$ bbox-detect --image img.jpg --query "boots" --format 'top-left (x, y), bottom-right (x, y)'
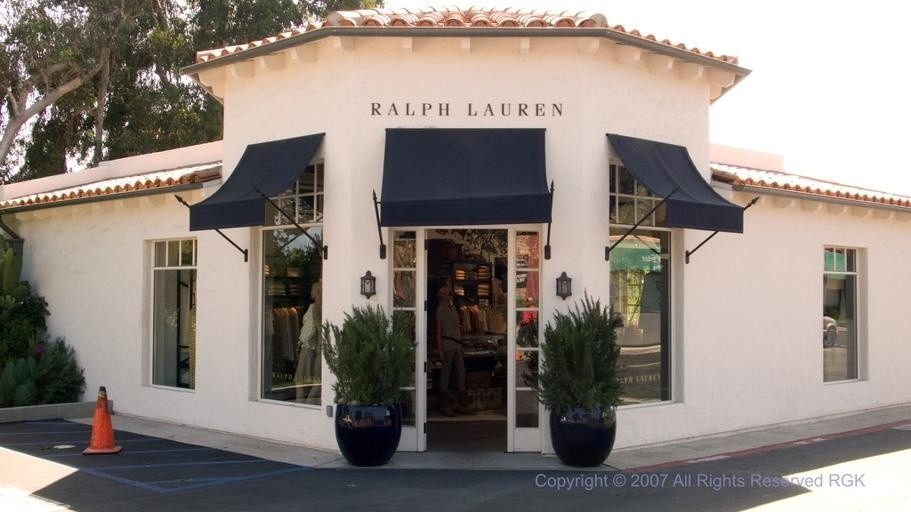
top-left (457, 391), bottom-right (479, 414)
top-left (440, 391), bottom-right (457, 417)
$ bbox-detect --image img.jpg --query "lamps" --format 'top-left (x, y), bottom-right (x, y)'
top-left (556, 271), bottom-right (573, 301)
top-left (359, 270), bottom-right (376, 301)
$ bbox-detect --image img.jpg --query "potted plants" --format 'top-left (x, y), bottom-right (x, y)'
top-left (321, 305), bottom-right (418, 466)
top-left (535, 288), bottom-right (625, 466)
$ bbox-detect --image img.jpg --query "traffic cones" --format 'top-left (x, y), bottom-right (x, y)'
top-left (78, 382), bottom-right (124, 457)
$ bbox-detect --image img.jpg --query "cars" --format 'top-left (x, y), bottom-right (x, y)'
top-left (822, 311), bottom-right (839, 351)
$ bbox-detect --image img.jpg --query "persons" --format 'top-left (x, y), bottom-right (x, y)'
top-left (293, 282), bottom-right (322, 403)
top-left (434, 286), bottom-right (477, 418)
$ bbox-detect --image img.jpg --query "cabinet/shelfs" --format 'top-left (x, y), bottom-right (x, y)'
top-left (262, 253), bottom-right (311, 307)
top-left (453, 258), bottom-right (493, 310)
